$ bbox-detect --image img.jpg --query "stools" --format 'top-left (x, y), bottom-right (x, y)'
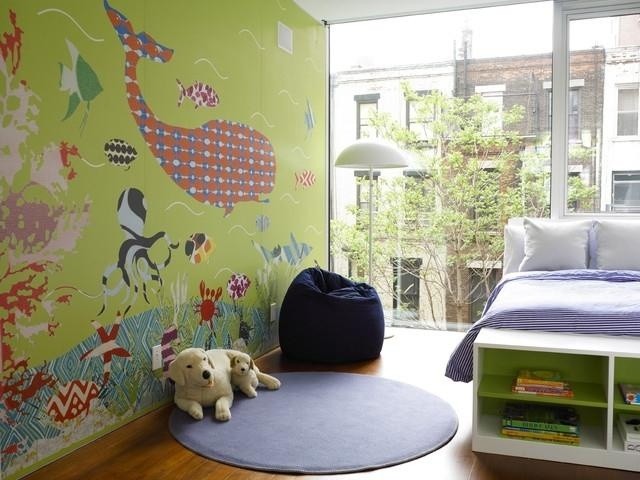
top-left (278, 267), bottom-right (385, 366)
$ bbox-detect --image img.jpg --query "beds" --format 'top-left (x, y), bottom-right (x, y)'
top-left (444, 217), bottom-right (639, 385)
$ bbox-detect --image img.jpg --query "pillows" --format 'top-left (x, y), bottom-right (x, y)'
top-left (518, 217), bottom-right (596, 272)
top-left (589, 218), bottom-right (640, 271)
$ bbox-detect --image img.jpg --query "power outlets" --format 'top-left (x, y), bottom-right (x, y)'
top-left (151, 344), bottom-right (162, 371)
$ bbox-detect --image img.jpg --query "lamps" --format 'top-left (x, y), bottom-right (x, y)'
top-left (333, 142), bottom-right (410, 285)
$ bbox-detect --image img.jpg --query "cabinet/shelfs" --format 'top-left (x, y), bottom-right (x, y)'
top-left (470, 327), bottom-right (495, 468)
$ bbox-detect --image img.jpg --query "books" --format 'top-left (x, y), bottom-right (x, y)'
top-left (615, 383), bottom-right (640, 453)
top-left (499, 367), bottom-right (583, 447)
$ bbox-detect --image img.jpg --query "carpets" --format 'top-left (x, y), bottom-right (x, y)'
top-left (166, 370), bottom-right (459, 475)
